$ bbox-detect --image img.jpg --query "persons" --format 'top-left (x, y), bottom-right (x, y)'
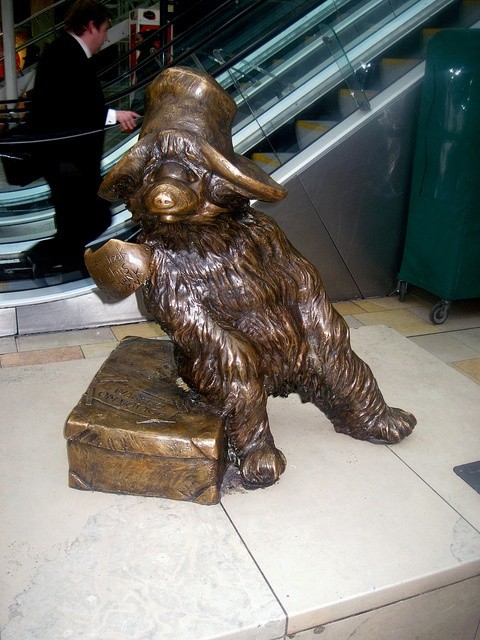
top-left (18, 1), bottom-right (143, 287)
top-left (41, 42), bottom-right (51, 54)
top-left (22, 44), bottom-right (41, 70)
top-left (130, 29), bottom-right (163, 130)
top-left (89, 43), bottom-right (120, 81)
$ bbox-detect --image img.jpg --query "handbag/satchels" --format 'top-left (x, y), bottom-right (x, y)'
top-left (0, 119), bottom-right (44, 186)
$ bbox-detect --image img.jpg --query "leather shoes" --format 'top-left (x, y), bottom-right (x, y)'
top-left (18, 251), bottom-right (49, 289)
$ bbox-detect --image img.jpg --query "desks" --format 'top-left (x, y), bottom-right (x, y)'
top-left (1, 324), bottom-right (479, 640)
